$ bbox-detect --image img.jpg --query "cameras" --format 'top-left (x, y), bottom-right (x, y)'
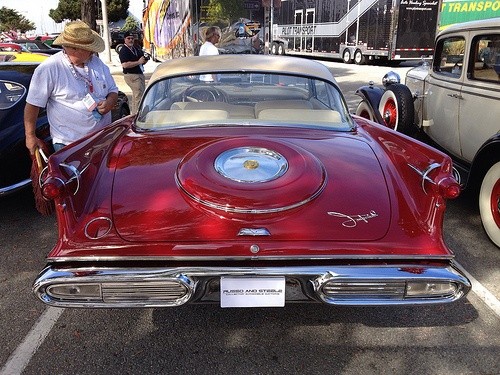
top-left (143, 53), bottom-right (150, 60)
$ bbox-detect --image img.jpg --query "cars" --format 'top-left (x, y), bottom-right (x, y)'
top-left (352, 17), bottom-right (500, 251)
top-left (0, 36), bottom-right (130, 200)
top-left (29, 52), bottom-right (472, 311)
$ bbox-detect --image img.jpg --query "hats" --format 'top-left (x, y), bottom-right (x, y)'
top-left (53, 21), bottom-right (105, 52)
top-left (124, 30), bottom-right (134, 38)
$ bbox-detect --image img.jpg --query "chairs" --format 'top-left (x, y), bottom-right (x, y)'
top-left (474, 68), bottom-right (500, 83)
top-left (257, 108), bottom-right (342, 123)
top-left (145, 110), bottom-right (230, 123)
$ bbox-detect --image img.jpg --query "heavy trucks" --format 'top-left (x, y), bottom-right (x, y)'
top-left (257, 0), bottom-right (443, 65)
top-left (143, 0), bottom-right (264, 63)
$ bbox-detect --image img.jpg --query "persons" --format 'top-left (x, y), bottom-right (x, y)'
top-left (199, 26), bottom-right (221, 84)
top-left (119, 30), bottom-right (150, 116)
top-left (24, 18), bottom-right (119, 178)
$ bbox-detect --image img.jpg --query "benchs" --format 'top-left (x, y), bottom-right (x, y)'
top-left (171, 100), bottom-right (313, 120)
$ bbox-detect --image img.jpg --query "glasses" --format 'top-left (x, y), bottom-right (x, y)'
top-left (127, 38), bottom-right (134, 40)
top-left (215, 34), bottom-right (219, 36)
